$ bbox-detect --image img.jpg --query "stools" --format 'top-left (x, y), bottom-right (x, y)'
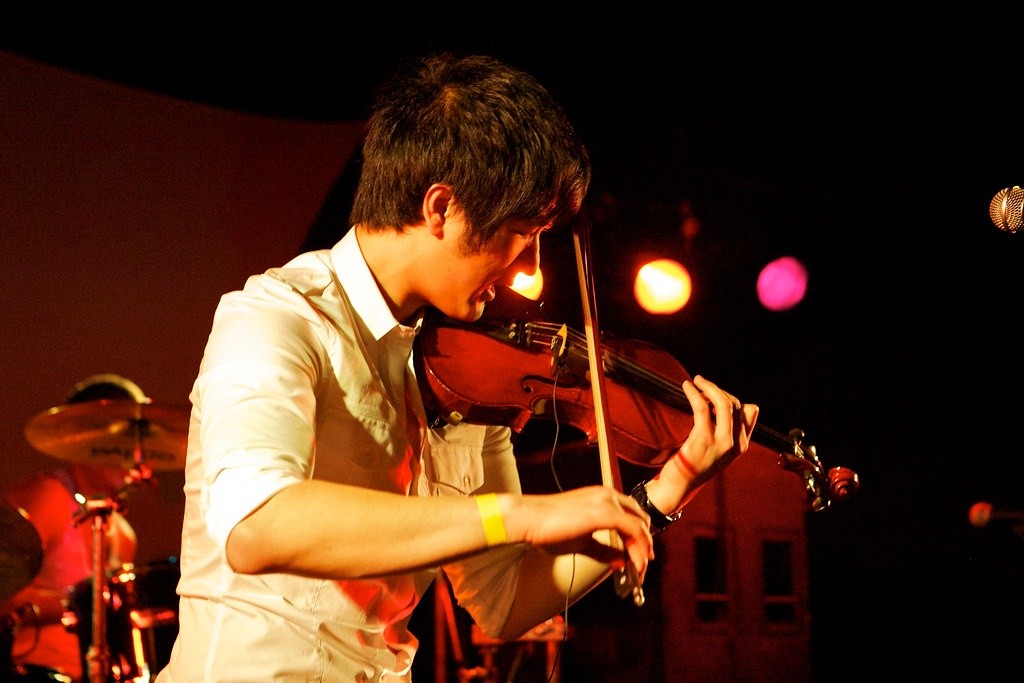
top-left (471, 623), bottom-right (573, 683)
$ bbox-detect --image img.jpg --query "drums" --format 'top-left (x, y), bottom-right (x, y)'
top-left (67, 554), bottom-right (182, 683)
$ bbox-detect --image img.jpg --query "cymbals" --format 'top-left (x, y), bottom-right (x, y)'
top-left (0, 496), bottom-right (44, 606)
top-left (24, 398), bottom-right (197, 472)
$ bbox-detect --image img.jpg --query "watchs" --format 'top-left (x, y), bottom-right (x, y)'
top-left (630, 480), bottom-right (683, 532)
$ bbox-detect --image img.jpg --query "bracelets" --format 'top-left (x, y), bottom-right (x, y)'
top-left (474, 492), bottom-right (506, 549)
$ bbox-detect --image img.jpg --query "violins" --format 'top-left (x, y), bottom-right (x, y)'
top-left (416, 286), bottom-right (865, 514)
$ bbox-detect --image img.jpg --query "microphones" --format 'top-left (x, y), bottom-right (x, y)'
top-left (990, 186), bottom-right (1024, 233)
top-left (968, 503), bottom-right (1024, 526)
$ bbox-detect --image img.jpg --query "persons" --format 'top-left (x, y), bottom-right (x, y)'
top-left (152, 52), bottom-right (759, 683)
top-left (0, 374), bottom-right (146, 683)
